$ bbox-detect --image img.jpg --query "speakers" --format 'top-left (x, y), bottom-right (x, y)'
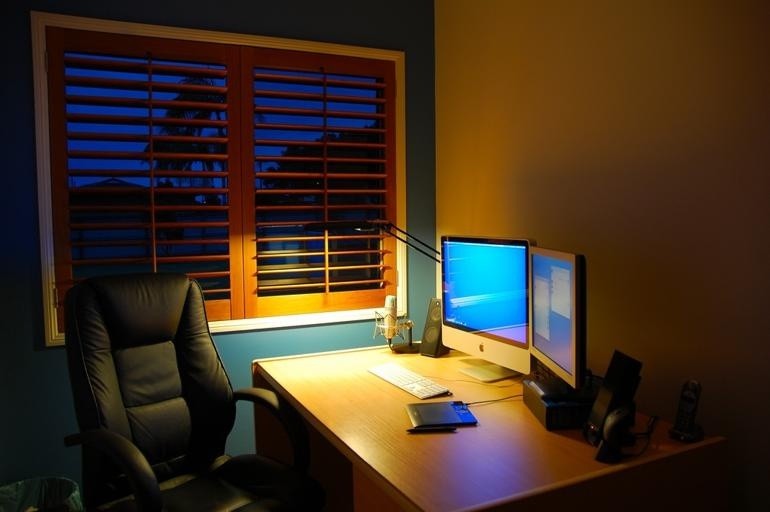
top-left (586, 350), bottom-right (643, 444)
top-left (420, 298), bottom-right (449, 357)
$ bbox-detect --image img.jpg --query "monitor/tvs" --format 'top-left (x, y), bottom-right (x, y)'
top-left (529, 247), bottom-right (589, 399)
top-left (441, 236), bottom-right (536, 382)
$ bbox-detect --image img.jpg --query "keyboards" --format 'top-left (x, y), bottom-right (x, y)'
top-left (369, 363), bottom-right (449, 399)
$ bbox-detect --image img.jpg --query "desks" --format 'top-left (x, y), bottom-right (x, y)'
top-left (251, 343), bottom-right (726, 511)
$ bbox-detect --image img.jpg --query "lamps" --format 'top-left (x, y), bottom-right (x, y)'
top-left (307, 223), bottom-right (440, 264)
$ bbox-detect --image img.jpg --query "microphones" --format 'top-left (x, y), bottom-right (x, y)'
top-left (385, 295), bottom-right (397, 339)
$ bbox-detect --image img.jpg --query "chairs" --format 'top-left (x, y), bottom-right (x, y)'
top-left (64, 276), bottom-right (325, 512)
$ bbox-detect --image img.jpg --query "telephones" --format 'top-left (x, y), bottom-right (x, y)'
top-left (668, 379), bottom-right (706, 442)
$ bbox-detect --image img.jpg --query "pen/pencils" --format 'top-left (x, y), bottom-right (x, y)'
top-left (406, 427), bottom-right (457, 433)
